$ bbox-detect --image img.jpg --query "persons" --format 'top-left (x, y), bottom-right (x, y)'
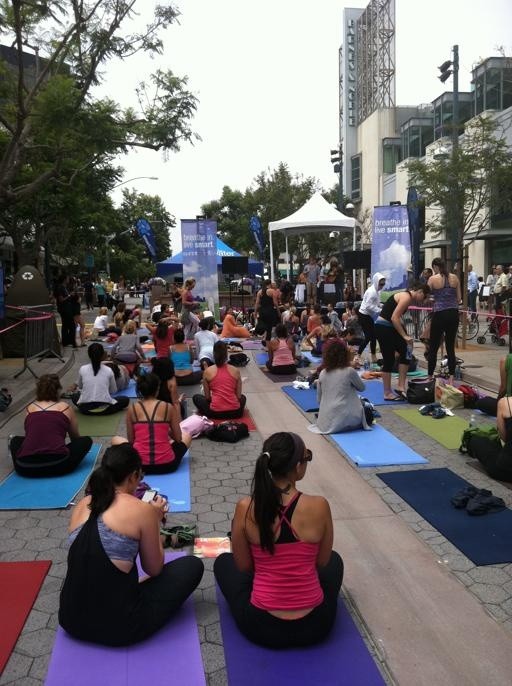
top-left (213, 431), bottom-right (344, 649)
top-left (52, 256), bottom-right (512, 483)
top-left (10, 374), bottom-right (92, 478)
top-left (58, 442), bottom-right (204, 647)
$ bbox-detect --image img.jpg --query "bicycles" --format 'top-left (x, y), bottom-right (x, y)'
top-left (420, 305), bottom-right (479, 341)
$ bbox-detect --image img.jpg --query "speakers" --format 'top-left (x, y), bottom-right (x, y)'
top-left (222, 256), bottom-right (249, 274)
top-left (338, 251), bottom-right (371, 268)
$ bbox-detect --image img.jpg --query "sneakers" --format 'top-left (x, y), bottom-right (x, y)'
top-left (160, 535), bottom-right (170, 549)
top-left (449, 485), bottom-right (478, 510)
top-left (466, 489), bottom-right (505, 515)
top-left (171, 536), bottom-right (184, 548)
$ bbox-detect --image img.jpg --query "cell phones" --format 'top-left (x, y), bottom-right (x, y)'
top-left (141, 490), bottom-right (157, 503)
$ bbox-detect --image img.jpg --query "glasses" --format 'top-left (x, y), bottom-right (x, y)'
top-left (380, 283), bottom-right (385, 286)
top-left (138, 472), bottom-right (145, 480)
top-left (302, 448), bottom-right (312, 462)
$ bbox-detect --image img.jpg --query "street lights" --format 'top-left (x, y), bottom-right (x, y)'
top-left (438, 46), bottom-right (461, 260)
top-left (106, 219), bottom-right (166, 280)
top-left (328, 201), bottom-right (356, 268)
top-left (102, 175), bottom-right (158, 284)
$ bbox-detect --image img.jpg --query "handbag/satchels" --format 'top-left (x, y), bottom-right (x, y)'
top-left (179, 414), bottom-right (214, 439)
top-left (435, 382), bottom-right (464, 409)
top-left (228, 353), bottom-right (250, 367)
top-left (180, 311), bottom-right (190, 325)
top-left (207, 421), bottom-right (249, 443)
top-left (361, 397), bottom-right (376, 426)
top-left (324, 283), bottom-right (336, 293)
top-left (459, 423), bottom-right (501, 453)
top-left (407, 378), bottom-right (436, 404)
top-left (457, 384), bottom-right (479, 409)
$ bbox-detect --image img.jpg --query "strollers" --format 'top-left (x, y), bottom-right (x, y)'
top-left (477, 305), bottom-right (510, 346)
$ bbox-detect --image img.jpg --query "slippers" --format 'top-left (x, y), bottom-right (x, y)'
top-left (384, 397), bottom-right (402, 401)
top-left (394, 390), bottom-right (408, 400)
